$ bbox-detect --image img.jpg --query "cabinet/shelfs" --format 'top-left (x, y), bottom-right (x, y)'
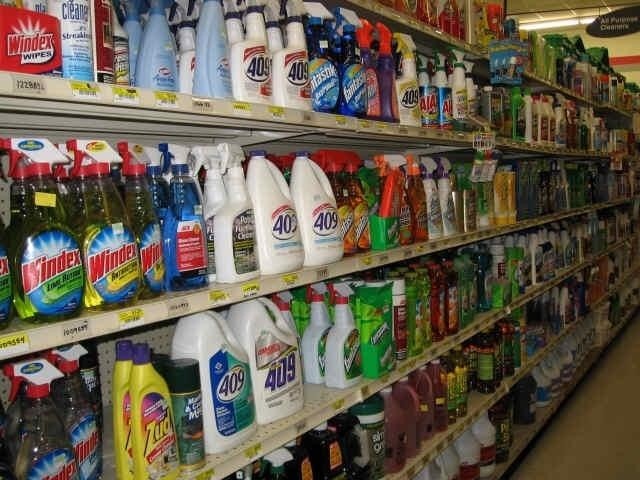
top-left (1, 1), bottom-right (639, 479)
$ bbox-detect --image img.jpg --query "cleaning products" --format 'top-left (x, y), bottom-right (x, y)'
top-left (0, 0), bottom-right (640, 480)
